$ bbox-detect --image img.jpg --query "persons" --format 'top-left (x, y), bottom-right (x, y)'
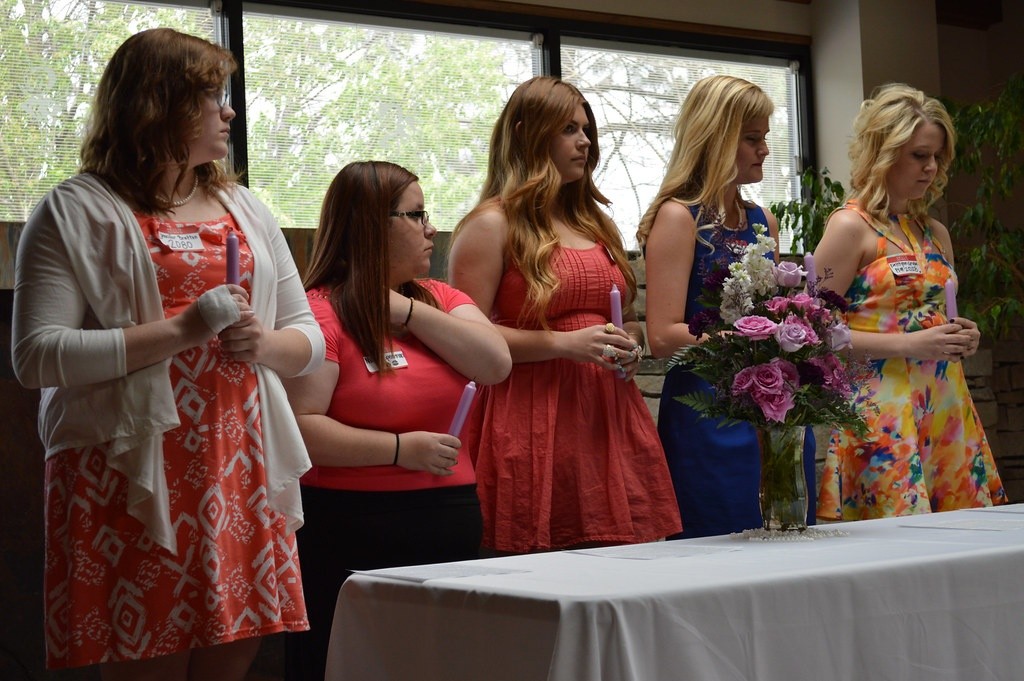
top-left (636, 76), bottom-right (816, 541)
top-left (813, 83), bottom-right (1009, 520)
top-left (448, 77), bottom-right (683, 554)
top-left (284, 161), bottom-right (512, 681)
top-left (11, 30), bottom-right (327, 681)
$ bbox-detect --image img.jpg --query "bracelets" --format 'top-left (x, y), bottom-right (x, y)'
top-left (393, 434), bottom-right (400, 465)
top-left (402, 297), bottom-right (415, 327)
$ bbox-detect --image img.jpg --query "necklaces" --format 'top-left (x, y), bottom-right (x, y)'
top-left (717, 196), bottom-right (743, 232)
top-left (154, 170), bottom-right (199, 206)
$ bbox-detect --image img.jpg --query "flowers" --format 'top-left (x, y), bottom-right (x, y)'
top-left (663, 221), bottom-right (875, 435)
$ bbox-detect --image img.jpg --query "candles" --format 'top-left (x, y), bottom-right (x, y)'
top-left (225, 225), bottom-right (240, 288)
top-left (944, 274), bottom-right (958, 320)
top-left (802, 246), bottom-right (817, 288)
top-left (325, 503), bottom-right (1023, 681)
top-left (608, 278), bottom-right (624, 331)
top-left (447, 375), bottom-right (477, 438)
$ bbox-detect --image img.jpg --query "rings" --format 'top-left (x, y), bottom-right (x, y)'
top-left (602, 345), bottom-right (615, 358)
top-left (634, 346), bottom-right (644, 363)
top-left (605, 323), bottom-right (615, 333)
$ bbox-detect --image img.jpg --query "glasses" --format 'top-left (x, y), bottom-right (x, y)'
top-left (214, 85), bottom-right (233, 108)
top-left (389, 210), bottom-right (430, 226)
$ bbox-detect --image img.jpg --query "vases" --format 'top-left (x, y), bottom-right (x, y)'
top-left (753, 423), bottom-right (811, 532)
